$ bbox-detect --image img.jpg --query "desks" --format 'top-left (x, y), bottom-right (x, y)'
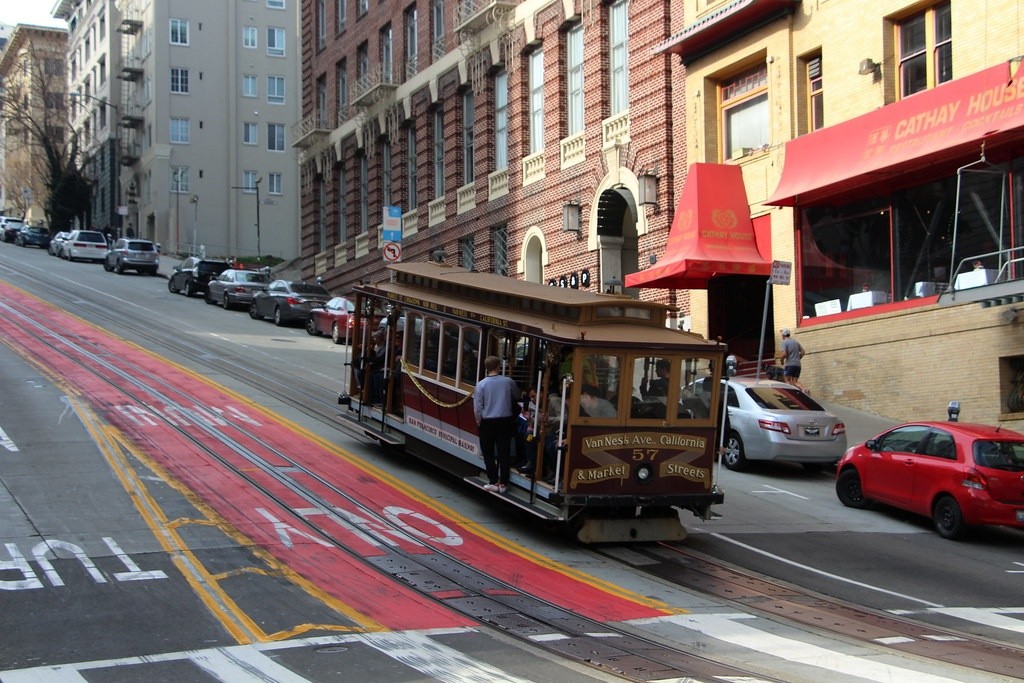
top-left (909, 281), bottom-right (948, 299)
top-left (954, 269), bottom-right (1006, 290)
top-left (847, 291), bottom-right (888, 311)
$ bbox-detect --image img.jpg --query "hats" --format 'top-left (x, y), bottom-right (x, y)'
top-left (782, 329), bottom-right (790, 336)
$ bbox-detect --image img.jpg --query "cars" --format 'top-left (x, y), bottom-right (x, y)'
top-left (834, 420), bottom-right (1024, 538)
top-left (47, 229), bottom-right (108, 262)
top-left (676, 375), bottom-right (849, 471)
top-left (168, 256), bottom-right (423, 345)
top-left (104, 238), bottom-right (160, 274)
top-left (15, 225), bottom-right (51, 248)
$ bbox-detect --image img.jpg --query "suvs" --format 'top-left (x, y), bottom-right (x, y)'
top-left (0, 215), bottom-right (25, 242)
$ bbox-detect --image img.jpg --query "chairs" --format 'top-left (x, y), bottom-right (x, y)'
top-left (933, 439), bottom-right (955, 463)
top-left (934, 281), bottom-right (949, 294)
top-left (886, 289), bottom-right (896, 303)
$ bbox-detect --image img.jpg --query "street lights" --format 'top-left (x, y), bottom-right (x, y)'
top-left (69, 91), bottom-right (120, 228)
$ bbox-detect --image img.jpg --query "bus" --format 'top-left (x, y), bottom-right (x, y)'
top-left (338, 249), bottom-right (738, 545)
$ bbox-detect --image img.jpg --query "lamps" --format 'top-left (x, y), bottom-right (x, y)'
top-left (637, 169), bottom-right (658, 207)
top-left (857, 58), bottom-right (880, 75)
top-left (563, 199), bottom-right (580, 232)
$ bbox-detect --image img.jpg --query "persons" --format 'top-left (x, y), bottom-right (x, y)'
top-left (127, 223), bottom-right (135, 238)
top-left (640, 359), bottom-right (670, 402)
top-left (473, 356), bottom-right (526, 494)
top-left (90, 223), bottom-right (118, 245)
top-left (354, 328), bottom-right (405, 412)
top-left (518, 385), bottom-right (568, 478)
top-left (779, 328), bottom-right (812, 397)
top-left (37, 219), bottom-right (43, 226)
top-left (555, 386), bottom-right (571, 490)
top-left (430, 329), bottom-right (477, 381)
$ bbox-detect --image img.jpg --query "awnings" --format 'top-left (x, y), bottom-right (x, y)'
top-left (625, 162), bottom-right (775, 291)
top-left (648, 0), bottom-right (804, 70)
top-left (765, 55), bottom-right (1024, 209)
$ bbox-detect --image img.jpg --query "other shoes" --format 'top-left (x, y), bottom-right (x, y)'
top-left (483, 483), bottom-right (499, 491)
top-left (805, 389), bottom-right (810, 395)
top-left (521, 460), bottom-right (563, 489)
top-left (499, 485), bottom-right (507, 494)
